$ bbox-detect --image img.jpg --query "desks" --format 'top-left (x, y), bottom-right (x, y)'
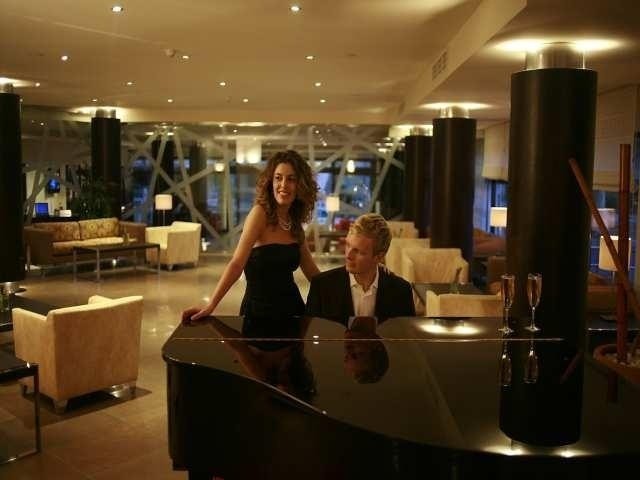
top-left (0, 350), bottom-right (42, 467)
top-left (318, 230), bottom-right (348, 261)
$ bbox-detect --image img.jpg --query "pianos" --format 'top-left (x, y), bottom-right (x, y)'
top-left (161, 316), bottom-right (640, 476)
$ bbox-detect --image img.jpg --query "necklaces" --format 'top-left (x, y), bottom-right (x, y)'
top-left (275, 209), bottom-right (293, 233)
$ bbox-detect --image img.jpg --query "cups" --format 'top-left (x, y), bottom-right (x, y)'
top-left (498, 275), bottom-right (515, 335)
top-left (524, 338), bottom-right (539, 385)
top-left (498, 339), bottom-right (513, 388)
top-left (525, 274), bottom-right (543, 332)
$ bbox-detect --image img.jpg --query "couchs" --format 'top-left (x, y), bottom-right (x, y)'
top-left (386, 221), bottom-right (418, 238)
top-left (385, 238), bottom-right (430, 276)
top-left (424, 289), bottom-right (505, 318)
top-left (401, 247), bottom-right (468, 285)
top-left (11, 293), bottom-right (143, 418)
top-left (144, 220), bottom-right (202, 272)
top-left (24, 216), bottom-right (148, 278)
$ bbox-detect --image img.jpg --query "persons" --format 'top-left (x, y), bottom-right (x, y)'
top-left (179, 317), bottom-right (319, 408)
top-left (181, 149), bottom-right (320, 316)
top-left (305, 213), bottom-right (416, 317)
top-left (317, 317), bottom-right (399, 385)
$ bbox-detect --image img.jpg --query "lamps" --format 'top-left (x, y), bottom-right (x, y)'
top-left (325, 192), bottom-right (340, 212)
top-left (489, 207), bottom-right (507, 231)
top-left (154, 193), bottom-right (173, 226)
top-left (598, 236), bottom-right (631, 285)
